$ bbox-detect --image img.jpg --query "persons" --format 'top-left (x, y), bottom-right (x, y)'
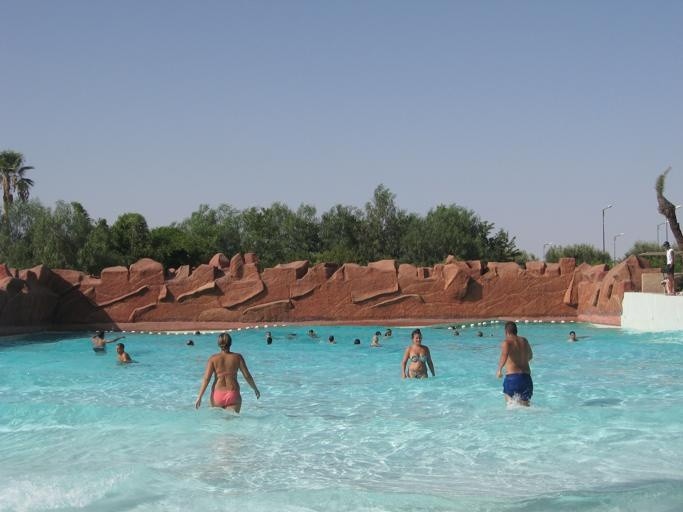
top-left (663, 240), bottom-right (676, 295)
top-left (400, 329), bottom-right (435, 378)
top-left (193, 333), bottom-right (260, 413)
top-left (375, 331), bottom-right (381, 336)
top-left (369, 334), bottom-right (382, 347)
top-left (353, 339), bottom-right (359, 344)
top-left (115, 343), bottom-right (132, 362)
top-left (328, 335), bottom-right (337, 344)
top-left (497, 320), bottom-right (534, 407)
top-left (384, 328), bottom-right (391, 336)
top-left (265, 330), bottom-right (271, 337)
top-left (266, 337), bottom-right (273, 346)
top-left (90, 329), bottom-right (125, 348)
top-left (308, 329), bottom-right (317, 337)
top-left (569, 330), bottom-right (576, 342)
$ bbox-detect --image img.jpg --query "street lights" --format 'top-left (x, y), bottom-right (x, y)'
top-left (542, 242), bottom-right (553, 261)
top-left (656, 221), bottom-right (669, 243)
top-left (666, 205), bottom-right (682, 243)
top-left (613, 232), bottom-right (624, 267)
top-left (602, 204), bottom-right (613, 252)
